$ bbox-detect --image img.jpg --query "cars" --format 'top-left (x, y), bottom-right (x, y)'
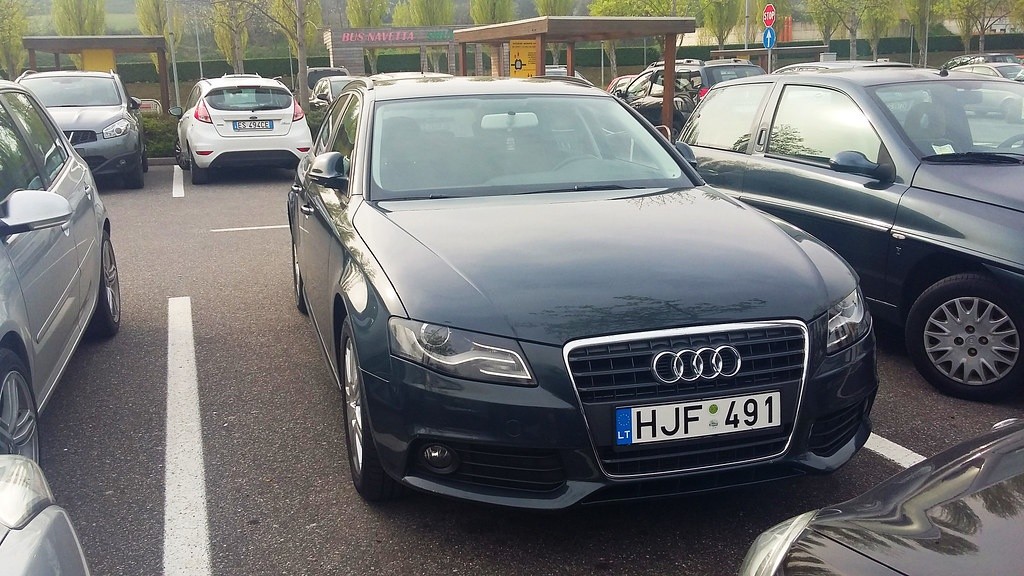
top-left (166, 74), bottom-right (314, 185)
top-left (545, 48), bottom-right (1024, 134)
top-left (308, 75), bottom-right (368, 122)
top-left (295, 76), bottom-right (880, 518)
top-left (1, 79), bottom-right (121, 469)
top-left (675, 63), bottom-right (1023, 402)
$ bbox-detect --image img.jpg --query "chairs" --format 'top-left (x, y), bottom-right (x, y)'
top-left (206, 90), bottom-right (229, 110)
top-left (905, 102), bottom-right (958, 156)
top-left (380, 117), bottom-right (439, 189)
top-left (256, 89), bottom-right (273, 107)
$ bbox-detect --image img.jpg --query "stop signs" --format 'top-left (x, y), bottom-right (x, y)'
top-left (762, 3), bottom-right (776, 28)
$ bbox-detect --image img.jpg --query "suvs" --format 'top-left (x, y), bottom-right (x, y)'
top-left (10, 70), bottom-right (148, 190)
top-left (294, 65), bottom-right (351, 105)
